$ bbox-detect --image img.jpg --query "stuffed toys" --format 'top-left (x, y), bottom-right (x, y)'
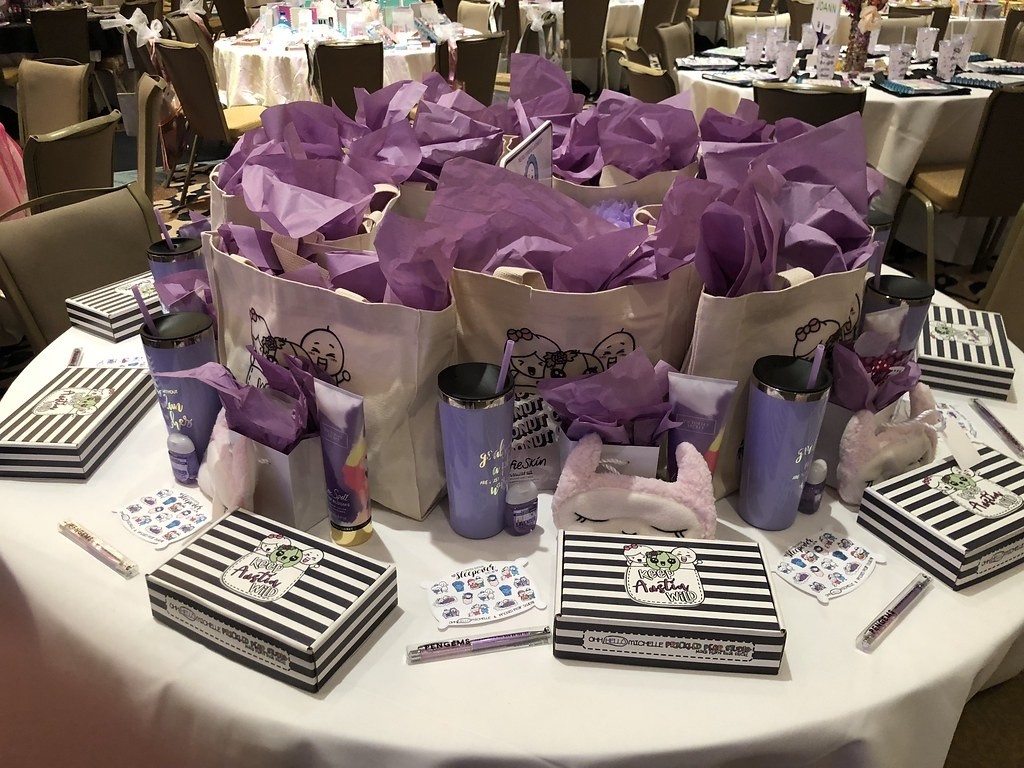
top-left (551, 434), bottom-right (718, 541)
top-left (836, 382), bottom-right (939, 505)
top-left (198, 407), bottom-right (256, 512)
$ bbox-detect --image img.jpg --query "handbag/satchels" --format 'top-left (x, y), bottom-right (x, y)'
top-left (201, 141), bottom-right (866, 533)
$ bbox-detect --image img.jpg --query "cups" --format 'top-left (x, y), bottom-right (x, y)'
top-left (138, 310), bottom-right (223, 465)
top-left (867, 209), bottom-right (893, 274)
top-left (738, 354), bottom-right (832, 531)
top-left (859, 276), bottom-right (936, 356)
top-left (438, 362), bottom-right (515, 539)
top-left (867, 27), bottom-right (974, 81)
top-left (744, 22), bottom-right (839, 79)
top-left (144, 237), bottom-right (205, 281)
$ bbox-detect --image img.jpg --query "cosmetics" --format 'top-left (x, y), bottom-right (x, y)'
top-left (69, 348), bottom-right (83, 366)
top-left (799, 460), bottom-right (827, 516)
top-left (167, 434), bottom-right (197, 482)
top-left (856, 572), bottom-right (931, 649)
top-left (969, 398), bottom-right (1024, 459)
top-left (505, 482), bottom-right (538, 537)
top-left (59, 520), bottom-right (137, 573)
top-left (408, 626), bottom-right (551, 664)
top-left (314, 378), bottom-right (373, 546)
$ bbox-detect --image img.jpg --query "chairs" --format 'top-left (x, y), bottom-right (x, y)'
top-left (1, 1), bottom-right (1024, 352)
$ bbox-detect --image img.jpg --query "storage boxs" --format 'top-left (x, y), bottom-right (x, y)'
top-left (65, 270), bottom-right (165, 344)
top-left (0, 366), bottom-right (159, 480)
top-left (856, 446), bottom-right (1023, 592)
top-left (913, 301), bottom-right (1016, 401)
top-left (146, 507), bottom-right (398, 694)
top-left (553, 529), bottom-right (786, 675)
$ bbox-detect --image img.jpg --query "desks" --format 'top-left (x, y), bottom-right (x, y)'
top-left (212, 20), bottom-right (485, 108)
top-left (0, 261), bottom-right (1023, 767)
top-left (674, 42), bottom-right (1024, 263)
top-left (457, 0), bottom-right (643, 90)
